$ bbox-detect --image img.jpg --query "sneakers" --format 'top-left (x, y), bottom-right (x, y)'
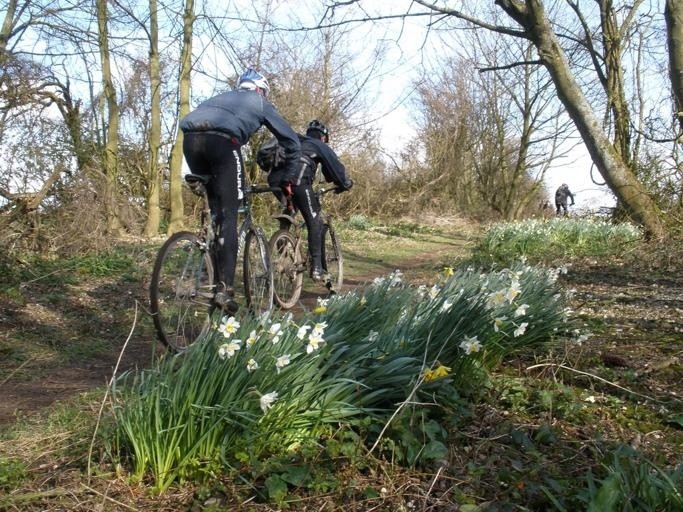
top-left (195, 228), bottom-right (216, 255)
top-left (311, 265), bottom-right (336, 283)
top-left (213, 280), bottom-right (238, 315)
top-left (276, 238), bottom-right (288, 257)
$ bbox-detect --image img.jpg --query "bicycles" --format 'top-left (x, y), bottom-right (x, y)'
top-left (146, 171), bottom-right (282, 356)
top-left (261, 183), bottom-right (346, 309)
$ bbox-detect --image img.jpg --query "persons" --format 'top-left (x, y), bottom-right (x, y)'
top-left (265, 119), bottom-right (353, 282)
top-left (176, 66), bottom-right (302, 316)
top-left (553, 183), bottom-right (574, 215)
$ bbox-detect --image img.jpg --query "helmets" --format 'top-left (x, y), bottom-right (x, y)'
top-left (305, 119), bottom-right (329, 143)
top-left (235, 67), bottom-right (271, 93)
